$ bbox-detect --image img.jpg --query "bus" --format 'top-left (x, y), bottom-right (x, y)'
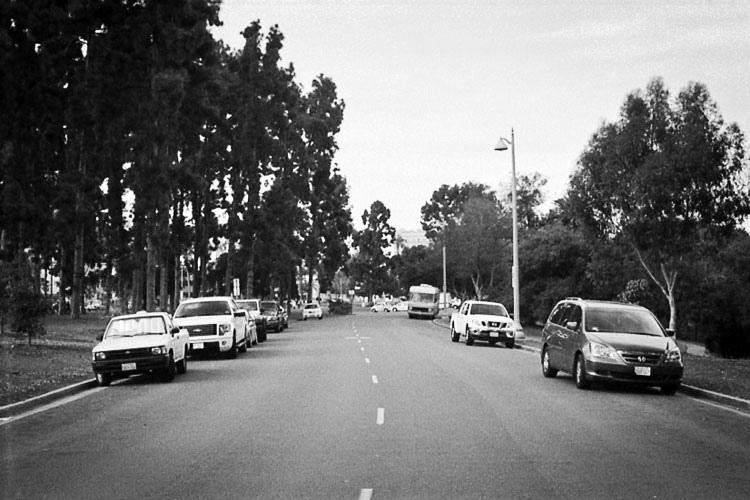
top-left (408, 283), bottom-right (440, 320)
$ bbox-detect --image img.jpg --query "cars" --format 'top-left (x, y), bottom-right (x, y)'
top-left (92, 311), bottom-right (190, 386)
top-left (542, 297), bottom-right (683, 395)
top-left (173, 297), bottom-right (289, 357)
top-left (297, 303), bottom-right (323, 320)
top-left (369, 301), bottom-right (408, 312)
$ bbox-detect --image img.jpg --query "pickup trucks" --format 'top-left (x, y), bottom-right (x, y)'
top-left (450, 300), bottom-right (515, 348)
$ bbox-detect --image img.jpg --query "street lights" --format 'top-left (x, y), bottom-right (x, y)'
top-left (496, 128), bottom-right (525, 331)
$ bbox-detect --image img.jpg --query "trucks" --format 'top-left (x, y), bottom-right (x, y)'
top-left (439, 293), bottom-right (462, 309)
